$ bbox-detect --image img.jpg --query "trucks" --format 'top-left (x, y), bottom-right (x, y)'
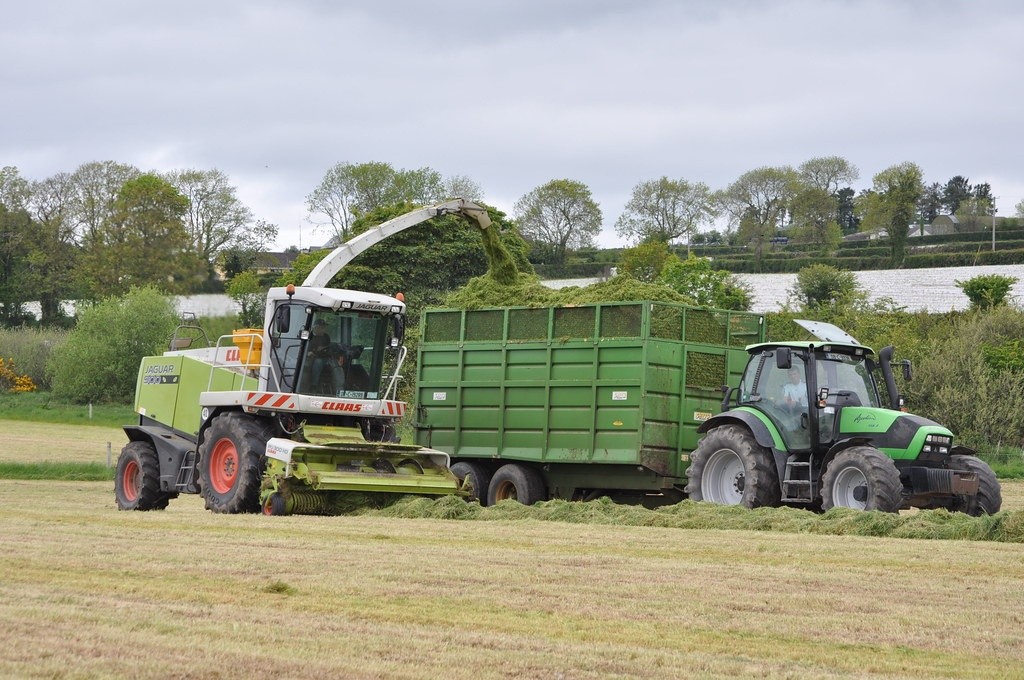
top-left (411, 297), bottom-right (1005, 517)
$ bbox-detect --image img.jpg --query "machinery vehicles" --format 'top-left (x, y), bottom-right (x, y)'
top-left (108, 196), bottom-right (493, 516)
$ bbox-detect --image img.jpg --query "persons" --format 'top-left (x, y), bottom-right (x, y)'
top-left (307, 319), bottom-right (346, 396)
top-left (780, 365), bottom-right (808, 408)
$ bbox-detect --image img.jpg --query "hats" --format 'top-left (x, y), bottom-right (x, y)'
top-left (314, 319), bottom-right (329, 326)
